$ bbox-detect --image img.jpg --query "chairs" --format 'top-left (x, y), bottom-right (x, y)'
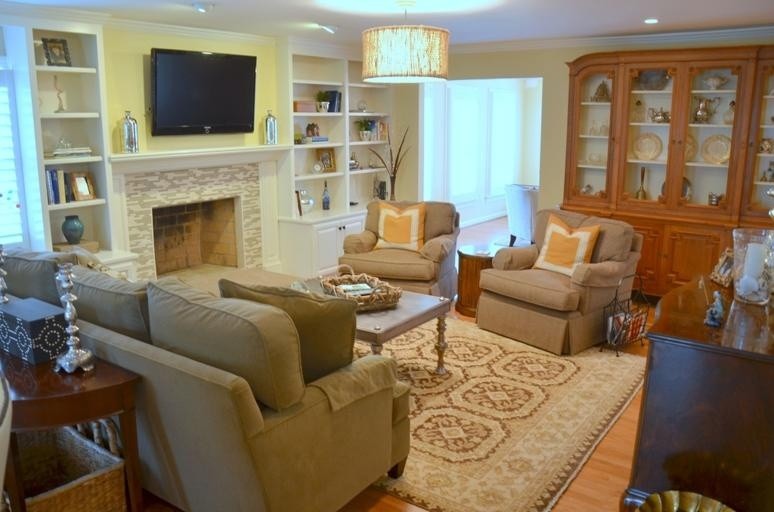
top-left (503, 185), bottom-right (540, 247)
top-left (474, 209), bottom-right (643, 357)
top-left (338, 199), bottom-right (459, 301)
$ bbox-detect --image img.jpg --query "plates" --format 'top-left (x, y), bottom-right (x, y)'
top-left (631, 131), bottom-right (664, 162)
top-left (701, 134), bottom-right (732, 165)
top-left (660, 177), bottom-right (695, 203)
top-left (683, 132), bottom-right (699, 164)
top-left (638, 69), bottom-right (670, 91)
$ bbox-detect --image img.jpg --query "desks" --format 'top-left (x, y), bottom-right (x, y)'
top-left (0, 349), bottom-right (144, 512)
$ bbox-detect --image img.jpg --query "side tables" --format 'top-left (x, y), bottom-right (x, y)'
top-left (455, 246), bottom-right (502, 318)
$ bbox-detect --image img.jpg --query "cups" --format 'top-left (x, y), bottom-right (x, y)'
top-left (708, 194), bottom-right (718, 205)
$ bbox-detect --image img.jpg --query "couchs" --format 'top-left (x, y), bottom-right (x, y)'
top-left (0, 245), bottom-right (410, 512)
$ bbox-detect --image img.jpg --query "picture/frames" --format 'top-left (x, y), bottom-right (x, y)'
top-left (42, 38), bottom-right (72, 67)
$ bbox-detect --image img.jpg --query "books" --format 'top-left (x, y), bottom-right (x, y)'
top-left (612, 305), bottom-right (649, 346)
top-left (44, 171), bottom-right (70, 204)
top-left (606, 310), bottom-right (626, 347)
top-left (324, 90), bottom-right (342, 113)
top-left (372, 179), bottom-right (386, 201)
top-left (339, 283), bottom-right (381, 297)
top-left (303, 136), bottom-right (330, 142)
top-left (366, 119), bottom-right (389, 141)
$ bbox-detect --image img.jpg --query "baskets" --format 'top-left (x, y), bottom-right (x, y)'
top-left (315, 270), bottom-right (404, 318)
top-left (2, 418), bottom-right (133, 512)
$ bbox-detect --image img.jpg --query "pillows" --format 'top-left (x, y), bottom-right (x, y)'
top-left (533, 209), bottom-right (634, 263)
top-left (372, 202), bottom-right (425, 252)
top-left (364, 200), bottom-right (457, 245)
top-left (531, 212), bottom-right (601, 278)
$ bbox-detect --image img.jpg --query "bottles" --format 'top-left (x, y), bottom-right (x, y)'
top-left (759, 137), bottom-right (773, 153)
top-left (296, 183), bottom-right (317, 214)
top-left (120, 111), bottom-right (139, 156)
top-left (321, 179), bottom-right (331, 211)
top-left (264, 109), bottom-right (279, 146)
top-left (723, 101), bottom-right (736, 124)
top-left (635, 166), bottom-right (647, 200)
top-left (306, 122), bottom-right (320, 137)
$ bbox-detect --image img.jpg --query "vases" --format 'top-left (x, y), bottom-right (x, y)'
top-left (117, 111), bottom-right (139, 154)
top-left (262, 110), bottom-right (277, 144)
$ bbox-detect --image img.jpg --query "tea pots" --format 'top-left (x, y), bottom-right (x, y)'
top-left (701, 75), bottom-right (728, 90)
top-left (692, 95), bottom-right (720, 123)
top-left (651, 106), bottom-right (672, 123)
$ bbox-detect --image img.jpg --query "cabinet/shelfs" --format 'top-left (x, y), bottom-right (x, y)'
top-left (11, 25), bottom-right (139, 284)
top-left (277, 36), bottom-right (396, 278)
top-left (619, 276), bottom-right (774, 512)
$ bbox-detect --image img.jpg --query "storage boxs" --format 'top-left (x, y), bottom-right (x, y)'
top-left (0, 297), bottom-right (71, 366)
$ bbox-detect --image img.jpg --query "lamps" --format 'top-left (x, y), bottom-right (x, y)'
top-left (362, 0), bottom-right (451, 84)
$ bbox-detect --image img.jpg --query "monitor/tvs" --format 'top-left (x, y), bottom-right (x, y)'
top-left (151, 47), bottom-right (258, 136)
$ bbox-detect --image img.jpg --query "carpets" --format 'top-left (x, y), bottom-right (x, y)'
top-left (353, 296), bottom-right (647, 512)
